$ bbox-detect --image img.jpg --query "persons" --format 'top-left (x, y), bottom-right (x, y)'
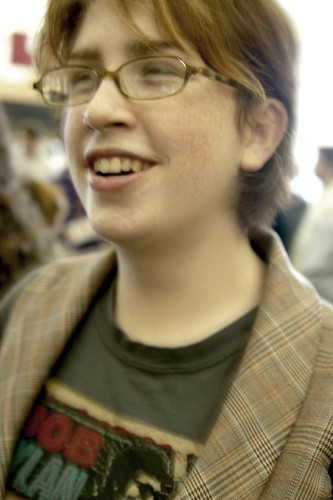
top-left (1, 0), bottom-right (333, 499)
top-left (286, 148), bottom-right (333, 302)
top-left (0, 122), bottom-right (75, 266)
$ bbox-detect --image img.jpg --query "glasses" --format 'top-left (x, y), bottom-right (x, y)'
top-left (33, 55), bottom-right (256, 105)
